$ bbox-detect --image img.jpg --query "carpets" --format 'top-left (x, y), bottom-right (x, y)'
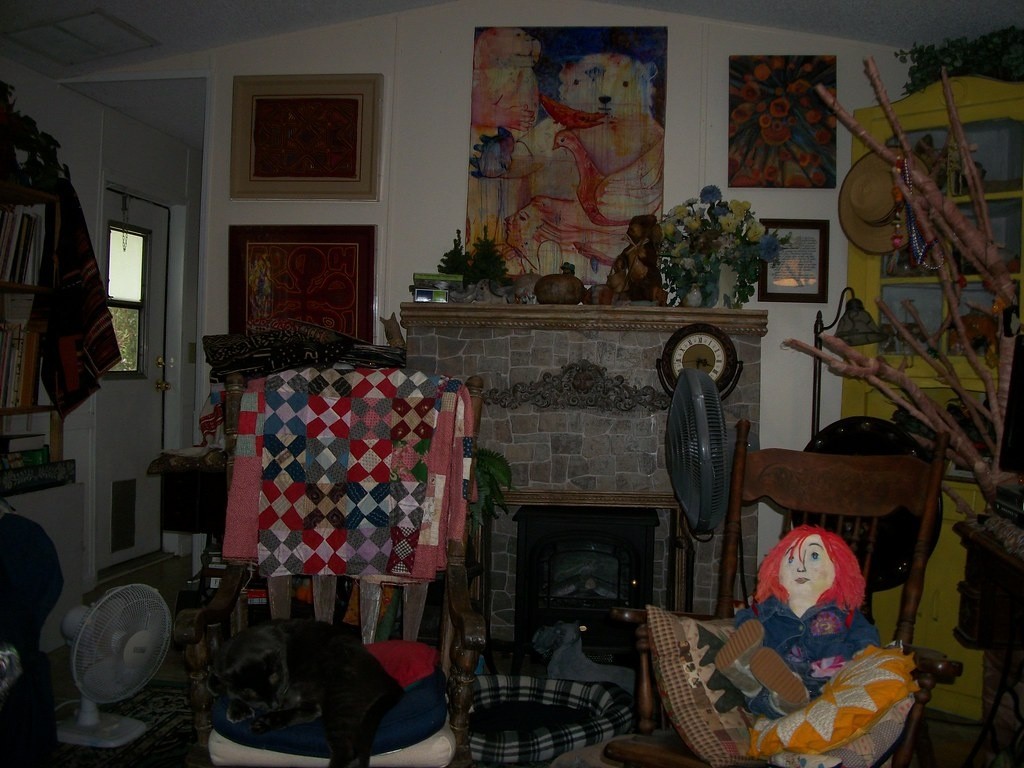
top-left (53, 679), bottom-right (202, 768)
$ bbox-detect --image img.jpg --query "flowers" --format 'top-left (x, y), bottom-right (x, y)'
top-left (658, 185), bottom-right (796, 309)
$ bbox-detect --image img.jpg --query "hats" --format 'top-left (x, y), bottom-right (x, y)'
top-left (837, 147), bottom-right (929, 256)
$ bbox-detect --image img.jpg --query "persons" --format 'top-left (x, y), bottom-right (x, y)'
top-left (716, 526), bottom-right (881, 719)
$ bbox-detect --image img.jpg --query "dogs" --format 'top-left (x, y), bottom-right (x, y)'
top-left (533, 621), bottom-right (636, 697)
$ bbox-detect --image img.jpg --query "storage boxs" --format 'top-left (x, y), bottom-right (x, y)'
top-left (415, 289), bottom-right (449, 303)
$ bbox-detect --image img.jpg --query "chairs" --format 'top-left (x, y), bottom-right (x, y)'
top-left (0, 512), bottom-right (67, 768)
top-left (174, 370), bottom-right (489, 767)
top-left (601, 414), bottom-right (951, 768)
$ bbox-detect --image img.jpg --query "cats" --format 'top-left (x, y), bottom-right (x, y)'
top-left (204, 616), bottom-right (400, 768)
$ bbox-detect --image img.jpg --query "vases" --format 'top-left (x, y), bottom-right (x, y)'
top-left (684, 283), bottom-right (707, 308)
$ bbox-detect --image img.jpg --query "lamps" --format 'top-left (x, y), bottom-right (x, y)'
top-left (813, 286), bottom-right (888, 439)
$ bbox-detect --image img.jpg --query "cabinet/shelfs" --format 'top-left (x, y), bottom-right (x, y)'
top-left (0, 180), bottom-right (77, 502)
top-left (511, 505), bottom-right (661, 670)
top-left (842, 77), bottom-right (1023, 728)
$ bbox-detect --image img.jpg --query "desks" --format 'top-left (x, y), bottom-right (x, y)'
top-left (951, 517), bottom-right (1023, 768)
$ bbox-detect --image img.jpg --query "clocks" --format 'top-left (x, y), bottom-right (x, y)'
top-left (655, 324), bottom-right (746, 400)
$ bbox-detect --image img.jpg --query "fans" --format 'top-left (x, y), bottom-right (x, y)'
top-left (665, 374), bottom-right (760, 615)
top-left (58, 584), bottom-right (172, 747)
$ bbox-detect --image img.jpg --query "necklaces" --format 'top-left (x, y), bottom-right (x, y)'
top-left (890, 155), bottom-right (945, 270)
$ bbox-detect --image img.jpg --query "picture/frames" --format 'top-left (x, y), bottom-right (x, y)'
top-left (229, 72), bottom-right (384, 204)
top-left (228, 224), bottom-right (376, 344)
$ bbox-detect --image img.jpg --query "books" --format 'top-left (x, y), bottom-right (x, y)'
top-left (0, 434), bottom-right (49, 470)
top-left (0, 321), bottom-right (42, 406)
top-left (0, 202), bottom-right (44, 284)
